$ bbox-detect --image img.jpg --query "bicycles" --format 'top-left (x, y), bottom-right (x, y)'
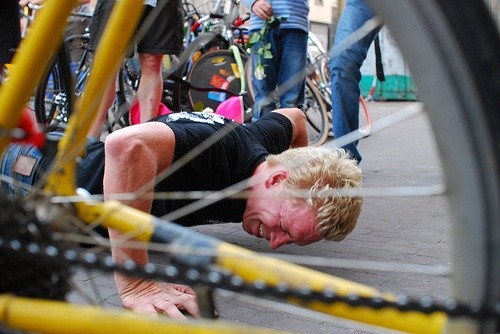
top-left (26, 3), bottom-right (372, 148)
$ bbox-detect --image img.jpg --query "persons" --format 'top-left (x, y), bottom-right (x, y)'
top-left (0, 0), bottom-right (387, 164)
top-left (0, 107), bottom-right (363, 321)
